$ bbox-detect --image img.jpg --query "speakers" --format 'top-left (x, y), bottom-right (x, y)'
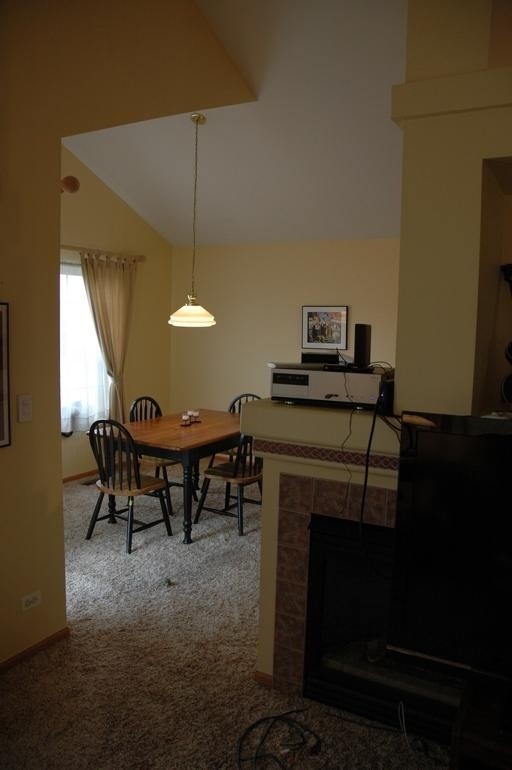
top-left (354, 323), bottom-right (371, 367)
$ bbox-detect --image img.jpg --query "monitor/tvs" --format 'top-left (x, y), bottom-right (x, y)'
top-left (379, 410), bottom-right (512, 709)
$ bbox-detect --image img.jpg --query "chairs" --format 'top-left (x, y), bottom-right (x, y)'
top-left (129, 396), bottom-right (199, 515)
top-left (193, 433), bottom-right (264, 537)
top-left (85, 419), bottom-right (173, 553)
top-left (193, 393), bottom-right (262, 494)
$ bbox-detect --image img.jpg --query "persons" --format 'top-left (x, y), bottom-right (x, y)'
top-left (312, 316), bottom-right (326, 342)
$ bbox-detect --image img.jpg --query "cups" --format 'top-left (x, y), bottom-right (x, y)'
top-left (182, 407), bottom-right (200, 426)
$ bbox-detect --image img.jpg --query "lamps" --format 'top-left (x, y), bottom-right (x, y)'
top-left (167, 113), bottom-right (217, 328)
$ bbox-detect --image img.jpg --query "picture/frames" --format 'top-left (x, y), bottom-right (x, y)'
top-left (0, 303), bottom-right (11, 448)
top-left (302, 306), bottom-right (348, 350)
